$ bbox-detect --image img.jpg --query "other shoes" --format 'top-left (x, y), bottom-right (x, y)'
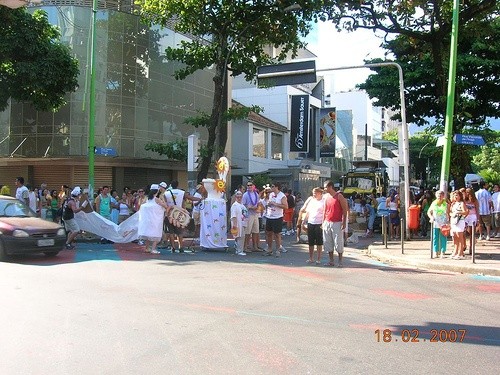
top-left (262, 251), bottom-right (273, 256)
top-left (434, 253), bottom-right (438, 258)
top-left (490, 233), bottom-right (497, 238)
top-left (441, 253), bottom-right (447, 258)
top-left (171, 247), bottom-right (186, 255)
top-left (280, 245), bottom-right (288, 252)
top-left (451, 253), bottom-right (457, 259)
top-left (275, 250), bottom-right (280, 257)
top-left (306, 258), bottom-right (314, 263)
top-left (316, 260), bottom-right (321, 264)
top-left (325, 262), bottom-right (335, 265)
top-left (144, 248), bottom-right (160, 254)
top-left (458, 253), bottom-right (464, 260)
top-left (238, 253), bottom-right (246, 256)
top-left (495, 233), bottom-right (500, 238)
top-left (486, 235), bottom-right (490, 240)
top-left (479, 236), bottom-right (483, 240)
top-left (65, 242), bottom-right (75, 249)
top-left (253, 248), bottom-right (263, 251)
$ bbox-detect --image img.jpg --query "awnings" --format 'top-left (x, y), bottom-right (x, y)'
top-left (343, 186), bottom-right (375, 193)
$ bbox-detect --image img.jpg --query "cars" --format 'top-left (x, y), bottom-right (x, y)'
top-left (0, 194), bottom-right (67, 261)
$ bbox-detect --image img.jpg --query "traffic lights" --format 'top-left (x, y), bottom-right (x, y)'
top-left (187, 134), bottom-right (201, 172)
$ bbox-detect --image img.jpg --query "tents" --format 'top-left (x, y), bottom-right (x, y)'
top-left (449, 174), bottom-right (485, 192)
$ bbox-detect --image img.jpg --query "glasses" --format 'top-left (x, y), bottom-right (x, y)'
top-left (271, 186), bottom-right (278, 188)
top-left (247, 185), bottom-right (254, 187)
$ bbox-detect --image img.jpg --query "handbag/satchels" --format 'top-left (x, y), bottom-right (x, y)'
top-left (440, 224), bottom-right (451, 236)
top-left (390, 213), bottom-right (397, 218)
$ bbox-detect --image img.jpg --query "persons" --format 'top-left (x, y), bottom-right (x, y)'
top-left (427, 190), bottom-right (469, 259)
top-left (296, 180), bottom-right (348, 267)
top-left (15, 176), bottom-right (304, 256)
top-left (349, 181), bottom-right (500, 241)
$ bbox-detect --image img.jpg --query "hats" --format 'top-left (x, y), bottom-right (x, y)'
top-left (151, 184), bottom-right (159, 189)
top-left (160, 182), bottom-right (168, 189)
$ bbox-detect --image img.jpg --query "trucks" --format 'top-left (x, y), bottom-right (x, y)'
top-left (338, 159), bottom-right (389, 201)
